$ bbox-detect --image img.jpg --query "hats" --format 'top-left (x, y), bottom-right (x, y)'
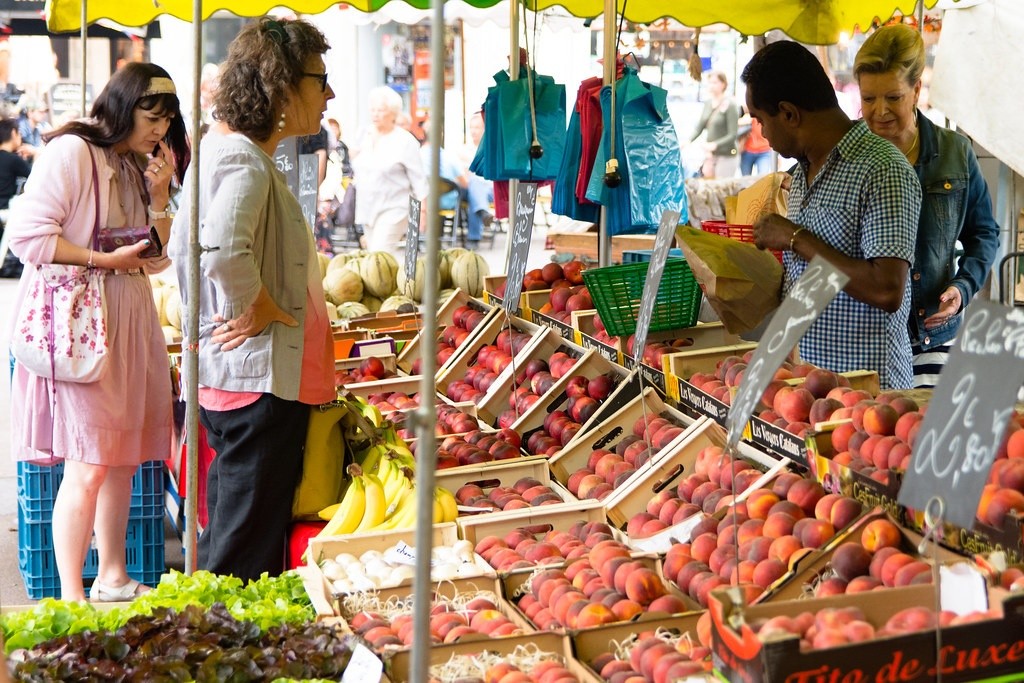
top-left (0, 83), bottom-right (25, 99)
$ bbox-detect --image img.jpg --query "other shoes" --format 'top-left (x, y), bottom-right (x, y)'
top-left (476, 210), bottom-right (493, 226)
top-left (464, 239), bottom-right (478, 251)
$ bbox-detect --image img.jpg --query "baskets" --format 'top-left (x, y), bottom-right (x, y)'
top-left (702, 219), bottom-right (784, 266)
top-left (579, 259), bottom-right (703, 336)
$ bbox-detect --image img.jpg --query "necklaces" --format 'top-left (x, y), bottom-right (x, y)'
top-left (904, 125), bottom-right (919, 156)
top-left (117, 182), bottom-right (128, 206)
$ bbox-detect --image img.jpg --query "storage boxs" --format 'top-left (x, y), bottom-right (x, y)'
top-left (16, 219), bottom-right (1024, 683)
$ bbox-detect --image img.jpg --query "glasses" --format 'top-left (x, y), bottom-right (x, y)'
top-left (32, 109), bottom-right (49, 113)
top-left (300, 73), bottom-right (328, 92)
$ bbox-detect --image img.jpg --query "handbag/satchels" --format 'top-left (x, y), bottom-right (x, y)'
top-left (9, 262), bottom-right (109, 382)
top-left (735, 170), bottom-right (791, 242)
top-left (291, 399), bottom-right (375, 521)
top-left (674, 225), bottom-right (785, 334)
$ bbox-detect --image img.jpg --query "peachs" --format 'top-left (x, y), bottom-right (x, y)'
top-left (338, 262), bottom-right (696, 473)
top-left (345, 598), bottom-right (585, 683)
top-left (477, 347), bottom-right (1023, 682)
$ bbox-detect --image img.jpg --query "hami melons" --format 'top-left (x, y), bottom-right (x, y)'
top-left (315, 247), bottom-right (490, 321)
top-left (148, 277), bottom-right (184, 349)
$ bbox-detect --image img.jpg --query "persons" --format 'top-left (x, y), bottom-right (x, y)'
top-left (167, 19), bottom-right (336, 589)
top-left (693, 73), bottom-right (738, 180)
top-left (200, 63), bottom-right (221, 140)
top-left (4, 61), bottom-right (192, 610)
top-left (739, 104), bottom-right (779, 176)
top-left (0, 49), bottom-right (53, 279)
top-left (740, 40), bottom-right (922, 391)
top-left (298, 87), bottom-right (495, 251)
top-left (854, 24), bottom-right (1001, 388)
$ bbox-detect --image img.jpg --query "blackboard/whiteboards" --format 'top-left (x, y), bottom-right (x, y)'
top-left (273, 136), bottom-right (319, 237)
top-left (501, 182), bottom-right (537, 313)
top-left (724, 255), bottom-right (851, 449)
top-left (896, 299), bottom-right (1024, 530)
top-left (403, 195), bottom-right (422, 281)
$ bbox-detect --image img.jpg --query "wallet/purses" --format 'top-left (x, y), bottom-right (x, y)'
top-left (97, 226), bottom-right (162, 258)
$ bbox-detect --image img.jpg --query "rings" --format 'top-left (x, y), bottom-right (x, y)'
top-left (223, 323), bottom-right (231, 332)
top-left (159, 161), bottom-right (166, 168)
top-left (154, 167), bottom-right (161, 174)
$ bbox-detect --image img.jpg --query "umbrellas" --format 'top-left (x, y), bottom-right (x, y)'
top-left (46, 1), bottom-right (938, 682)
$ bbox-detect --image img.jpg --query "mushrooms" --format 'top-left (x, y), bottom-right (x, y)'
top-left (319, 539), bottom-right (481, 592)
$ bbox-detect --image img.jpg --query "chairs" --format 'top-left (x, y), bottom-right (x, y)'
top-left (325, 182), bottom-right (363, 252)
top-left (418, 177), bottom-right (507, 250)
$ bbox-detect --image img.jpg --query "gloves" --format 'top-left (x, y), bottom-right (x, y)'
top-left (338, 182), bottom-right (356, 224)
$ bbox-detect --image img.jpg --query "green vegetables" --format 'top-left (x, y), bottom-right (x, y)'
top-left (0, 569), bottom-right (355, 683)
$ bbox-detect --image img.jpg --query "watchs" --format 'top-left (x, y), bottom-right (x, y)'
top-left (148, 203), bottom-right (170, 220)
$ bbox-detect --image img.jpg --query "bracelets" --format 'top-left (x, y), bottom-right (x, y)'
top-left (790, 228), bottom-right (806, 253)
top-left (87, 250), bottom-right (96, 268)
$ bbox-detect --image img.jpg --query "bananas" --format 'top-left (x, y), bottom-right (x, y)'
top-left (300, 383), bottom-right (458, 564)
